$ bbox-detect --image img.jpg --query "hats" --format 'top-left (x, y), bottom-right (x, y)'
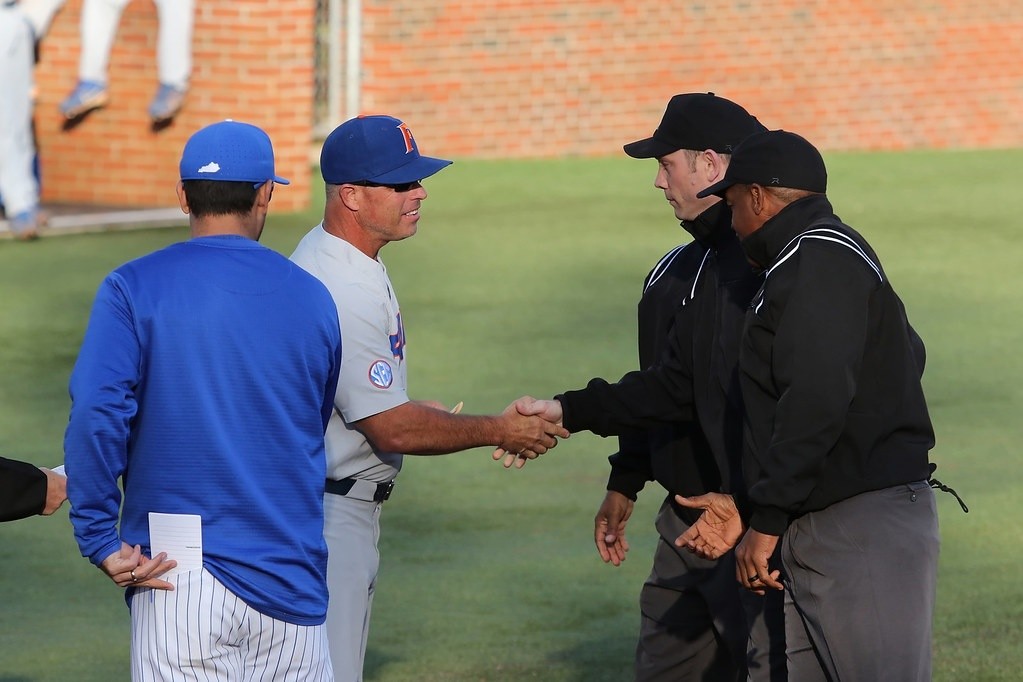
top-left (623, 92), bottom-right (770, 159)
top-left (179, 122), bottom-right (290, 185)
top-left (321, 115), bottom-right (453, 184)
top-left (696, 130), bottom-right (827, 199)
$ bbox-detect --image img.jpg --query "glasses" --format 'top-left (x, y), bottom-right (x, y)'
top-left (350, 179), bottom-right (421, 192)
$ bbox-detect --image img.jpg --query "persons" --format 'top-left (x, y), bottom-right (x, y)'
top-left (0, 457), bottom-right (67, 523)
top-left (64, 121), bottom-right (342, 682)
top-left (494, 91), bottom-right (937, 682)
top-left (287, 116), bottom-right (569, 682)
top-left (0, 1), bottom-right (192, 239)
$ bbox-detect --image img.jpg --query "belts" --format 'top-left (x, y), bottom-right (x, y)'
top-left (322, 477), bottom-right (396, 501)
